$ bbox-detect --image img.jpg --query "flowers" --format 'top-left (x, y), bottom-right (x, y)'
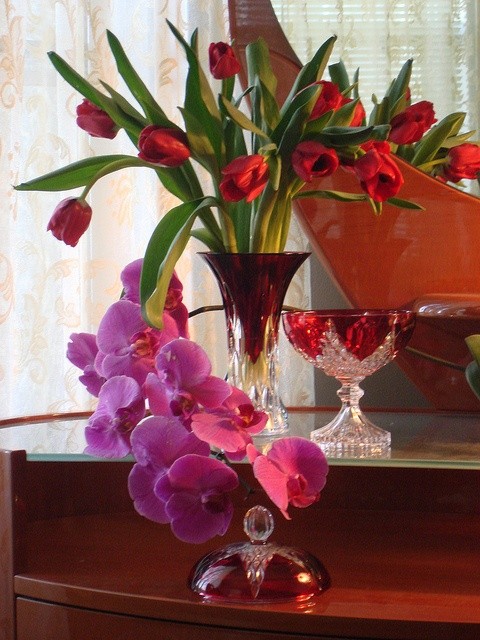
top-left (13, 16), bottom-right (480, 331)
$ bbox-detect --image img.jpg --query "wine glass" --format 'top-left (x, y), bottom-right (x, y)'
top-left (283, 308), bottom-right (413, 454)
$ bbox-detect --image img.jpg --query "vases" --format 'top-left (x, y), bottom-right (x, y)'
top-left (195, 248), bottom-right (313, 436)
